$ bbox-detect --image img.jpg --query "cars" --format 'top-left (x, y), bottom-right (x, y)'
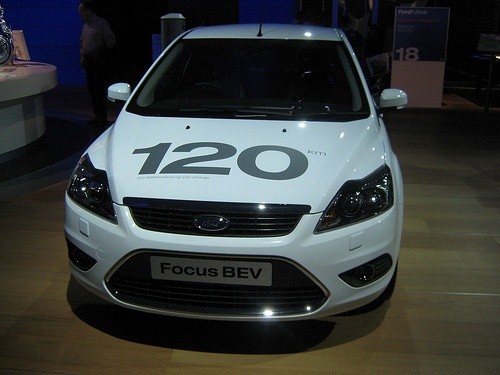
top-left (63, 25), bottom-right (408, 325)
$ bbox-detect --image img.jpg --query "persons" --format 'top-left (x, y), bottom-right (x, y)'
top-left (79, 2), bottom-right (118, 128)
